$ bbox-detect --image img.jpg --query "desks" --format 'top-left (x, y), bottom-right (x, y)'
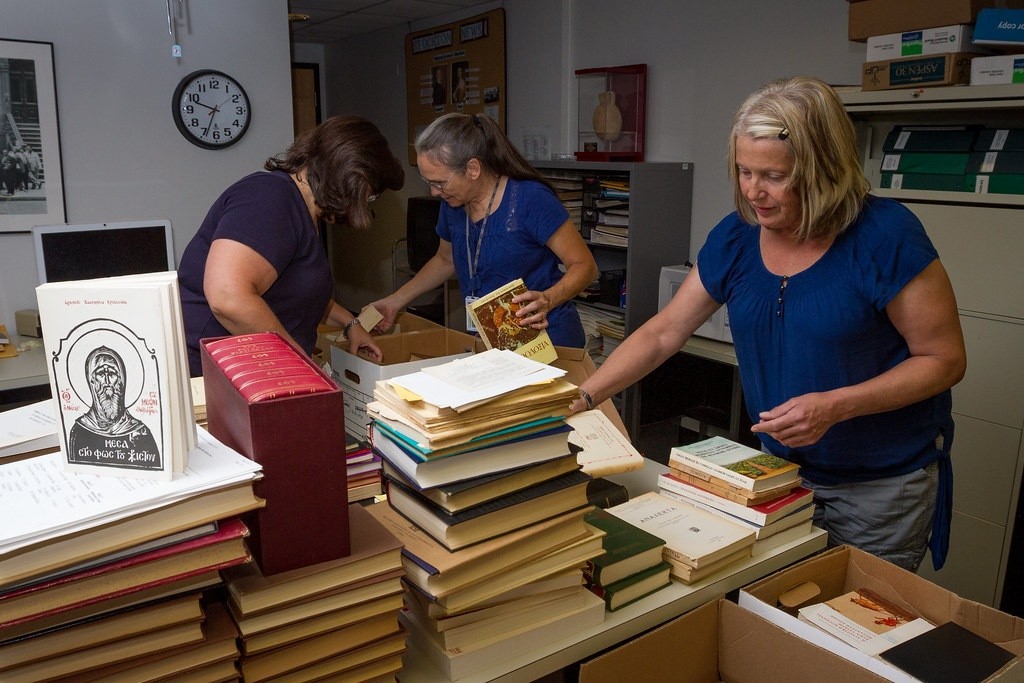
top-left (0, 333), bottom-right (51, 405)
top-left (396, 456), bottom-right (828, 683)
top-left (630, 336), bottom-right (743, 443)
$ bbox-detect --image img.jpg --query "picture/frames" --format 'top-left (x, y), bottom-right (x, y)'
top-left (0, 38), bottom-right (68, 235)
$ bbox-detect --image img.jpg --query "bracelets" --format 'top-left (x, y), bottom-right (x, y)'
top-left (578, 389), bottom-right (593, 410)
top-left (343, 319), bottom-right (360, 338)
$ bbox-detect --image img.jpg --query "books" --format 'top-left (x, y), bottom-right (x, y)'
top-left (0, 346), bottom-right (817, 683)
top-left (467, 278), bottom-right (558, 365)
top-left (34, 271), bottom-right (199, 481)
top-left (798, 586), bottom-right (1018, 683)
top-left (540, 171), bottom-right (630, 361)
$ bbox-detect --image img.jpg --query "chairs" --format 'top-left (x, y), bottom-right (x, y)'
top-left (393, 195), bottom-right (440, 293)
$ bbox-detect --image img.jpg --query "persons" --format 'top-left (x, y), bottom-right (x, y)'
top-left (557, 76), bottom-right (968, 572)
top-left (359, 113), bottom-right (599, 349)
top-left (176, 115), bottom-right (405, 379)
top-left (432, 69), bottom-right (447, 105)
top-left (453, 67), bottom-right (468, 103)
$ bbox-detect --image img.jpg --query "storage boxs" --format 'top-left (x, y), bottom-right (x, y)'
top-left (849, 0), bottom-right (1024, 92)
top-left (578, 544), bottom-right (1024, 683)
top-left (15, 308), bottom-right (42, 337)
top-left (311, 311), bottom-right (632, 446)
top-left (200, 331), bottom-right (350, 576)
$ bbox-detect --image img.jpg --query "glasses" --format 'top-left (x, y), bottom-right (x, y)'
top-left (365, 188), bottom-right (380, 202)
top-left (418, 166), bottom-right (467, 192)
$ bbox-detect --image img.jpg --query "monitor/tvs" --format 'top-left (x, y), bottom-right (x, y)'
top-left (32, 219), bottom-right (175, 283)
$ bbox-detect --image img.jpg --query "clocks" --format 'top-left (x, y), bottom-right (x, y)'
top-left (172, 69), bottom-right (252, 151)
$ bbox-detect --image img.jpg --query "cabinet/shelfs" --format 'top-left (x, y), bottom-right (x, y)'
top-left (825, 85), bottom-right (1024, 609)
top-left (527, 159), bottom-right (694, 442)
